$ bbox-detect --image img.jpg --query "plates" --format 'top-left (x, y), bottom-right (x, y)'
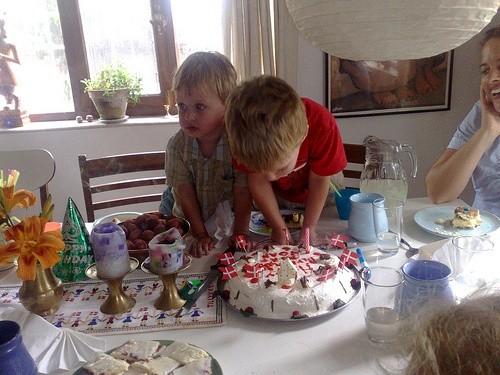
top-left (70, 339), bottom-right (223, 375)
top-left (248, 208), bottom-right (305, 235)
top-left (413, 206), bottom-right (500, 238)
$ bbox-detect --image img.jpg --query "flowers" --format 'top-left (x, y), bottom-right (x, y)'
top-left (0, 170), bottom-right (66, 282)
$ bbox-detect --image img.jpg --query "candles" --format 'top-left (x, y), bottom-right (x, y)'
top-left (90, 222), bottom-right (130, 276)
top-left (146, 228), bottom-right (186, 273)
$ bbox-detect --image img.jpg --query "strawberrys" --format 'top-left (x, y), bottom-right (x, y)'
top-left (119, 211), bottom-right (186, 248)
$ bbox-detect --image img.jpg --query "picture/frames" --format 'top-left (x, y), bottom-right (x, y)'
top-left (326, 48), bottom-right (454, 118)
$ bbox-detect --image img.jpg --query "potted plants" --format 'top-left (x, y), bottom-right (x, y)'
top-left (87, 66), bottom-right (136, 119)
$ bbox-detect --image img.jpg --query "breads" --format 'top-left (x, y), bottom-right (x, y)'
top-left (80, 340), bottom-right (215, 375)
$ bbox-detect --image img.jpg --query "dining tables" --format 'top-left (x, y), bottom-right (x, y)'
top-left (0, 198), bottom-right (500, 375)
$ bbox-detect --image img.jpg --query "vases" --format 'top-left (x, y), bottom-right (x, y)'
top-left (18, 262), bottom-right (65, 315)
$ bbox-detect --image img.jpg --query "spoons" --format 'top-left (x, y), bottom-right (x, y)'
top-left (400, 238), bottom-right (419, 258)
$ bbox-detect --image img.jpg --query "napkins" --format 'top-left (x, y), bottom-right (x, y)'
top-left (2, 304), bottom-right (107, 372)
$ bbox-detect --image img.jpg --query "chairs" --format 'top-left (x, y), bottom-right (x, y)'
top-left (77, 150), bottom-right (166, 223)
top-left (344, 142), bottom-right (384, 178)
top-left (0, 150), bottom-right (56, 212)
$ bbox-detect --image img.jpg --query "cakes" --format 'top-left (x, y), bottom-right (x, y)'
top-left (452, 206), bottom-right (481, 229)
top-left (220, 243), bottom-right (360, 319)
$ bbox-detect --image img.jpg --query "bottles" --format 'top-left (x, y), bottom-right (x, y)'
top-left (395, 259), bottom-right (458, 320)
top-left (1, 320), bottom-right (39, 374)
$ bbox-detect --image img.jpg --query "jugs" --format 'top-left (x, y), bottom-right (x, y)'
top-left (362, 134), bottom-right (418, 203)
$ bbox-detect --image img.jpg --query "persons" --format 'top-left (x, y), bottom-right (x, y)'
top-left (159, 50), bottom-right (252, 257)
top-left (405, 280), bottom-right (500, 375)
top-left (0, 20), bottom-right (21, 110)
top-left (424, 26), bottom-right (500, 219)
top-left (223, 74), bottom-right (348, 246)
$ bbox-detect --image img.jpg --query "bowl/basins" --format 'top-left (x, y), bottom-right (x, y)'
top-left (92, 212), bottom-right (140, 229)
top-left (117, 212), bottom-right (191, 268)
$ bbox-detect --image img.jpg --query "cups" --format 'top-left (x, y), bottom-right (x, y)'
top-left (372, 197), bottom-right (403, 254)
top-left (347, 192), bottom-right (388, 242)
top-left (450, 236), bottom-right (494, 284)
top-left (358, 266), bottom-right (406, 344)
top-left (334, 188), bottom-right (361, 220)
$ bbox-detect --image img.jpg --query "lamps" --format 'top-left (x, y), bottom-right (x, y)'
top-left (287, 0), bottom-right (500, 59)
